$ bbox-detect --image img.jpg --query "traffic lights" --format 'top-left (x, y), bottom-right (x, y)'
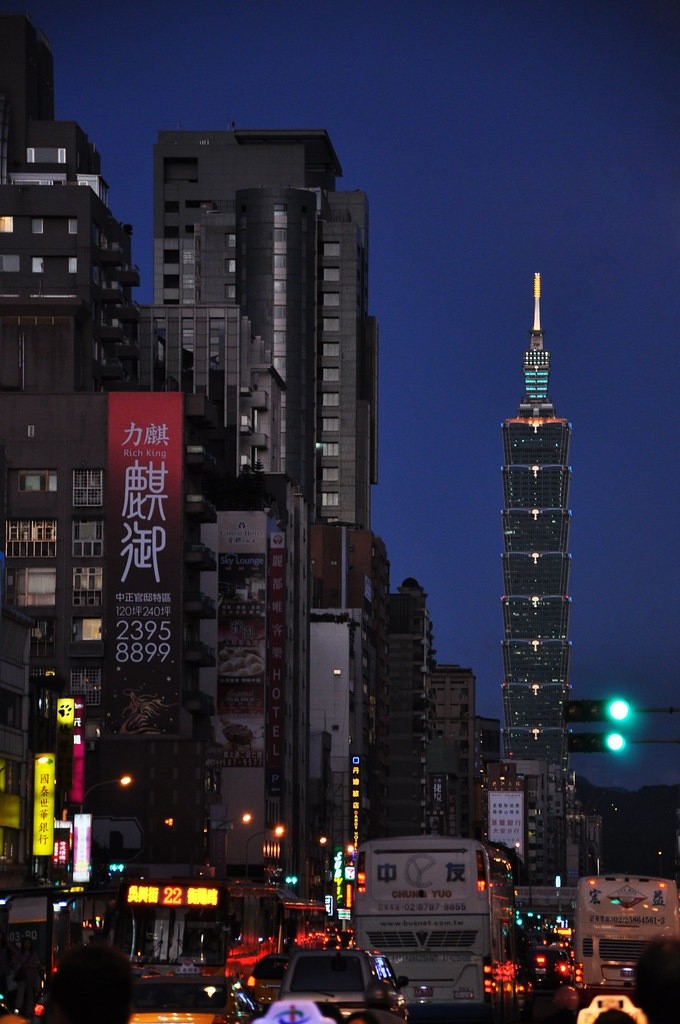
top-left (566, 697), bottom-right (634, 720)
top-left (569, 733), bottom-right (626, 752)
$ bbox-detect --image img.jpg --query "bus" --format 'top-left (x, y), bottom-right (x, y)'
top-left (353, 836), bottom-right (514, 1004)
top-left (575, 876), bottom-right (680, 989)
top-left (108, 879), bottom-right (330, 1024)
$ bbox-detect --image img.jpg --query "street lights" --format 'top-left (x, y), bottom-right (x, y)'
top-left (214, 812), bottom-right (252, 878)
top-left (80, 776), bottom-right (132, 926)
top-left (244, 822), bottom-right (285, 880)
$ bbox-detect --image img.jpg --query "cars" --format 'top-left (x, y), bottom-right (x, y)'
top-left (247, 947), bottom-right (409, 1024)
top-left (515, 949), bottom-right (575, 1018)
